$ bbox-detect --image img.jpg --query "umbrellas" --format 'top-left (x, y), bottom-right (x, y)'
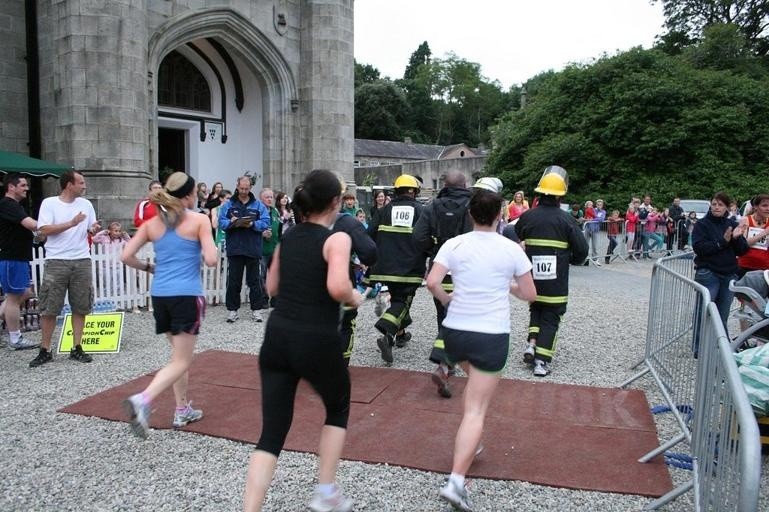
top-left (0, 149), bottom-right (76, 180)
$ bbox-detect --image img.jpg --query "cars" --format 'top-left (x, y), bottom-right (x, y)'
top-left (738, 200), bottom-right (752, 217)
top-left (678, 199), bottom-right (711, 220)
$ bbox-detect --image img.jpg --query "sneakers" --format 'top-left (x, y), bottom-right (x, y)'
top-left (378, 334), bottom-right (394, 363)
top-left (605, 257), bottom-right (609, 264)
top-left (441, 479), bottom-right (470, 510)
top-left (30, 348), bottom-right (53, 367)
top-left (308, 488), bottom-right (352, 511)
top-left (523, 343), bottom-right (552, 375)
top-left (228, 310), bottom-right (238, 322)
top-left (433, 366), bottom-right (452, 398)
top-left (123, 395), bottom-right (150, 439)
top-left (375, 296), bottom-right (386, 317)
top-left (0, 337), bottom-right (42, 351)
top-left (396, 333), bottom-right (410, 348)
top-left (252, 310), bottom-right (263, 322)
top-left (628, 247), bottom-right (664, 262)
top-left (69, 345), bottom-right (92, 362)
top-left (174, 406), bottom-right (202, 428)
top-left (594, 261), bottom-right (601, 266)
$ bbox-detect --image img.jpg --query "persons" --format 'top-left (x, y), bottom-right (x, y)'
top-left (239, 166), bottom-right (371, 511)
top-left (0, 172), bottom-right (45, 352)
top-left (91, 222), bottom-right (130, 314)
top-left (327, 166), bottom-right (699, 399)
top-left (135, 176), bottom-right (303, 323)
top-left (692, 192), bottom-right (769, 359)
top-left (29, 168), bottom-right (103, 368)
top-left (424, 197), bottom-right (537, 511)
top-left (121, 171), bottom-right (220, 441)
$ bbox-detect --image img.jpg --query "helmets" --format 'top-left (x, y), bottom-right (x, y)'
top-left (394, 173), bottom-right (421, 189)
top-left (533, 165), bottom-right (570, 196)
top-left (471, 174), bottom-right (503, 193)
